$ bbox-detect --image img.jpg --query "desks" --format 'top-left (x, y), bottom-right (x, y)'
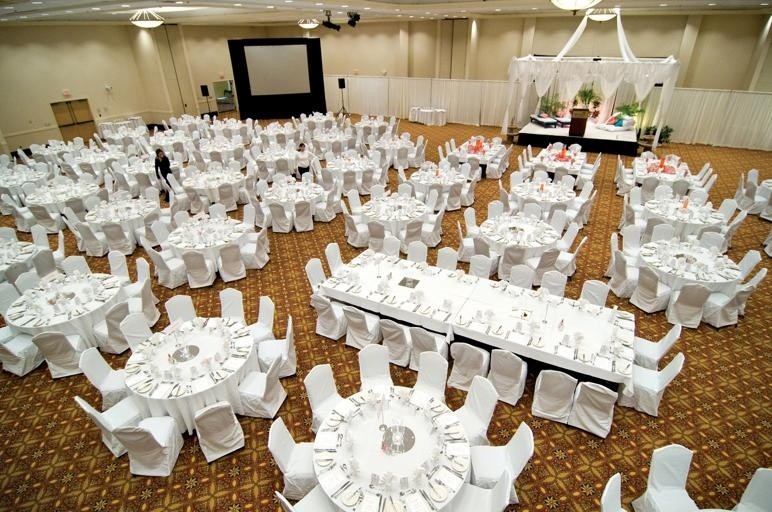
top-left (323, 250), bottom-right (637, 387)
top-left (314, 383), bottom-right (471, 512)
top-left (123, 317), bottom-right (257, 434)
top-left (4, 273), bottom-right (124, 339)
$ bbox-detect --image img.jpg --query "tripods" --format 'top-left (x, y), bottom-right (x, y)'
top-left (336, 89), bottom-right (351, 118)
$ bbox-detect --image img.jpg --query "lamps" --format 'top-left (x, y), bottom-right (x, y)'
top-left (199, 84), bottom-right (217, 125)
top-left (587, 6), bottom-right (617, 25)
top-left (347, 11), bottom-right (361, 28)
top-left (126, 9), bottom-right (168, 31)
top-left (336, 76), bottom-right (351, 120)
top-left (296, 17), bottom-right (321, 31)
top-left (322, 8), bottom-right (341, 32)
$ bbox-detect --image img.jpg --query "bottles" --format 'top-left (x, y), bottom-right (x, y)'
top-left (467, 138), bottom-right (494, 153)
top-left (347, 158), bottom-right (351, 165)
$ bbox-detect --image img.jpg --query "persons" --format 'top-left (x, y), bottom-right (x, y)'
top-left (154, 148), bottom-right (173, 202)
top-left (292, 142), bottom-right (310, 177)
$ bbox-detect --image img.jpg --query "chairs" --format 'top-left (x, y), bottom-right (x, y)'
top-left (118, 311), bottom-right (155, 355)
top-left (257, 315), bottom-right (298, 378)
top-left (237, 353), bottom-right (288, 419)
top-left (356, 343), bottom-right (395, 390)
top-left (31, 330), bottom-right (89, 380)
top-left (471, 421), bottom-right (535, 502)
top-left (78, 347), bottom-right (129, 410)
top-left (453, 374), bottom-right (499, 441)
top-left (380, 318), bottom-right (411, 368)
top-left (1, 326), bottom-right (19, 343)
top-left (126, 278), bottom-right (161, 329)
top-left (631, 443), bottom-right (701, 511)
top-left (597, 471), bottom-right (629, 511)
top-left (267, 416), bottom-right (317, 499)
top-left (219, 288), bottom-right (245, 320)
top-left (247, 296), bottom-right (276, 343)
top-left (72, 393), bottom-right (147, 460)
top-left (305, 258), bottom-right (327, 308)
top-left (311, 294), bottom-right (348, 340)
top-left (530, 370), bottom-right (579, 425)
top-left (92, 301), bottom-right (131, 356)
top-left (445, 471), bottom-right (511, 511)
top-left (409, 325), bottom-right (448, 373)
top-left (634, 323), bottom-right (683, 369)
top-left (412, 349), bottom-right (449, 401)
top-left (2, 332), bottom-right (45, 377)
top-left (303, 363), bottom-right (345, 424)
top-left (342, 305), bottom-right (380, 350)
top-left (268, 485), bottom-right (336, 511)
top-left (487, 348), bottom-right (528, 406)
top-left (164, 294), bottom-right (197, 325)
top-left (731, 468), bottom-right (772, 511)
top-left (458, 108), bottom-right (772, 329)
top-left (0, 108), bottom-right (517, 288)
top-left (193, 401), bottom-right (248, 463)
top-left (15, 271), bottom-right (41, 293)
top-left (618, 352), bottom-right (685, 416)
top-left (569, 380), bottom-right (619, 440)
top-left (448, 342), bottom-right (490, 393)
top-left (114, 416), bottom-right (184, 478)
top-left (123, 256), bottom-right (161, 303)
top-left (1, 284), bottom-right (20, 317)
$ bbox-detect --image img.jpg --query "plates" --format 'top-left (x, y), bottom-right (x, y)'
top-left (637, 153), bottom-right (738, 281)
top-left (363, 167), bottom-right (493, 222)
top-left (85, 198), bottom-right (156, 222)
top-left (7, 270), bottom-right (120, 327)
top-left (314, 387), bottom-right (466, 512)
top-left (482, 146), bottom-right (587, 248)
top-left (0, 239), bottom-right (34, 272)
top-left (167, 169), bottom-right (324, 249)
top-left (328, 251), bottom-right (636, 377)
top-left (124, 316), bottom-right (250, 398)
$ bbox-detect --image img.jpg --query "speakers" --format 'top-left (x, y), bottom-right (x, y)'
top-left (200, 85), bottom-right (209, 96)
top-left (338, 78), bottom-right (345, 89)
